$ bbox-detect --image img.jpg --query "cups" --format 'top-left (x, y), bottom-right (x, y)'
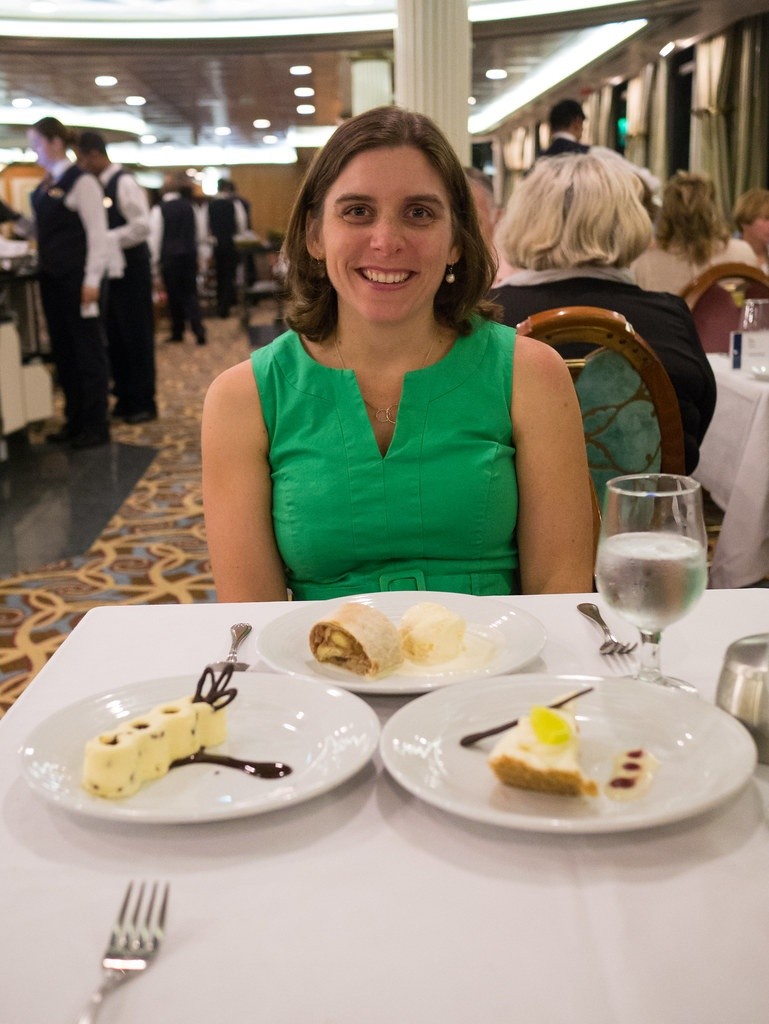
top-left (736, 298), bottom-right (769, 382)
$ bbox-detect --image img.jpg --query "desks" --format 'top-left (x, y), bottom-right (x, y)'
top-left (0, 590), bottom-right (769, 1024)
top-left (692, 348), bottom-right (769, 590)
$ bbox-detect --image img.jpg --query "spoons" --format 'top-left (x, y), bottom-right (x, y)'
top-left (206, 624), bottom-right (252, 672)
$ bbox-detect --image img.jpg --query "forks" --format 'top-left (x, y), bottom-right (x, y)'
top-left (576, 603), bottom-right (638, 655)
top-left (78, 877), bottom-right (172, 1024)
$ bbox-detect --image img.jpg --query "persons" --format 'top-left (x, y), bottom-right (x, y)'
top-left (27, 116), bottom-right (112, 450)
top-left (492, 147), bottom-right (716, 477)
top-left (539, 99), bottom-right (593, 156)
top-left (149, 178), bottom-right (251, 343)
top-left (461, 163), bottom-right (514, 285)
top-left (199, 106), bottom-right (595, 602)
top-left (74, 130), bottom-right (160, 425)
top-left (626, 170), bottom-right (769, 297)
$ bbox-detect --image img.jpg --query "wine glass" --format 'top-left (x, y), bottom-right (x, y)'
top-left (592, 473), bottom-right (709, 698)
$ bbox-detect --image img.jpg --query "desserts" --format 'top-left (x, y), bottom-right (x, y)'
top-left (80, 664), bottom-right (239, 800)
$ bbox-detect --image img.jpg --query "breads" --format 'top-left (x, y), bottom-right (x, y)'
top-left (487, 705), bottom-right (585, 798)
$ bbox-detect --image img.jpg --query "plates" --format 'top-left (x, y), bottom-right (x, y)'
top-left (17, 669), bottom-right (381, 826)
top-left (378, 673), bottom-right (758, 835)
top-left (255, 590), bottom-right (550, 697)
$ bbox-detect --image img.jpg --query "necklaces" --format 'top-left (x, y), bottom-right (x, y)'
top-left (332, 328), bottom-right (440, 427)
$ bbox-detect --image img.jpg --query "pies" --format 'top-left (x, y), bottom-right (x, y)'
top-left (309, 602), bottom-right (411, 679)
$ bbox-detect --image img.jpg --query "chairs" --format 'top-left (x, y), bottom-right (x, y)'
top-left (517, 307), bottom-right (686, 544)
top-left (679, 261), bottom-right (769, 356)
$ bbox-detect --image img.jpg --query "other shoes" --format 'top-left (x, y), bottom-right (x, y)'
top-left (45, 427), bottom-right (109, 448)
top-left (166, 337), bottom-right (182, 343)
top-left (111, 405), bottom-right (157, 425)
top-left (196, 326), bottom-right (205, 344)
